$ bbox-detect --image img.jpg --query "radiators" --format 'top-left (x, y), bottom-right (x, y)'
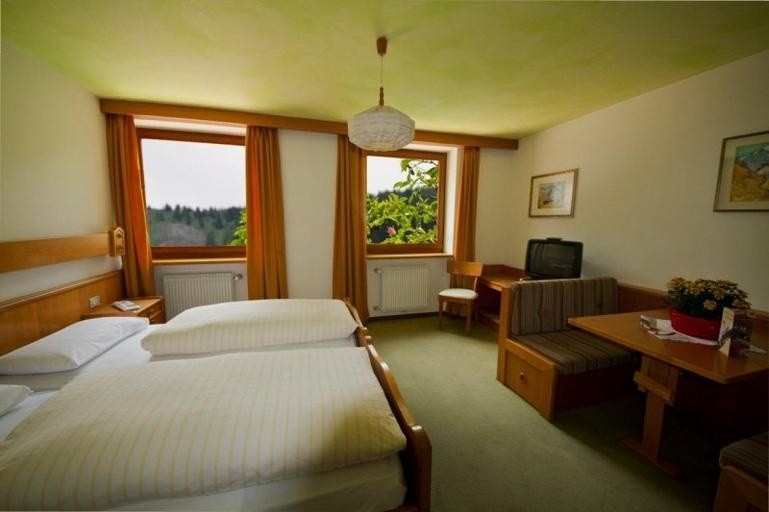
top-left (162, 272), bottom-right (242, 322)
top-left (374, 265), bottom-right (433, 313)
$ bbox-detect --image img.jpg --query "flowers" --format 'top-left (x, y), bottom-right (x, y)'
top-left (666, 277), bottom-right (752, 320)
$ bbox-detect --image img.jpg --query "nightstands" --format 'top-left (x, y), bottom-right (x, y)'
top-left (81, 295), bottom-right (167, 324)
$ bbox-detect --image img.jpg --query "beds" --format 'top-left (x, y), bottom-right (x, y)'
top-left (1, 269), bottom-right (432, 512)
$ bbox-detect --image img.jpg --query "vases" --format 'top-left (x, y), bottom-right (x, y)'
top-left (668, 309), bottom-right (720, 341)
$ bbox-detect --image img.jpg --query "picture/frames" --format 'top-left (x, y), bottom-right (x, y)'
top-left (528, 168), bottom-right (579, 218)
top-left (713, 130), bottom-right (769, 212)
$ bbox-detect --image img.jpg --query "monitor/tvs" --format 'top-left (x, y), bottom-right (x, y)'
top-left (524, 238), bottom-right (584, 279)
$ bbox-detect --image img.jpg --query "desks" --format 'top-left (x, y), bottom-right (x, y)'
top-left (478, 274), bottom-right (535, 331)
top-left (567, 308), bottom-right (768, 477)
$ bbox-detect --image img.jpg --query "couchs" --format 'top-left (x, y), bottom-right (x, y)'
top-left (715, 432), bottom-right (768, 509)
top-left (496, 276), bottom-right (642, 422)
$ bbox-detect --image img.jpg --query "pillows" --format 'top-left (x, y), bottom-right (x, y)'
top-left (1, 383), bottom-right (33, 416)
top-left (0, 315), bottom-right (150, 375)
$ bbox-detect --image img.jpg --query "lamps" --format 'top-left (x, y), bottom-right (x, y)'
top-left (348, 37), bottom-right (416, 152)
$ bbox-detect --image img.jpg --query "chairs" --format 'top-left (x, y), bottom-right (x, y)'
top-left (437, 259), bottom-right (484, 335)
